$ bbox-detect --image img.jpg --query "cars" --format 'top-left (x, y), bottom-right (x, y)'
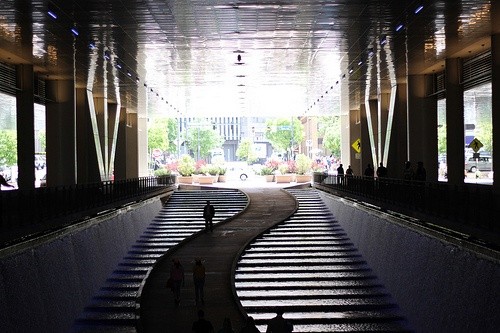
top-left (35, 155), bottom-right (46, 170)
top-left (0, 164), bottom-right (12, 182)
top-left (465, 155), bottom-right (492, 172)
top-left (39, 174), bottom-right (46, 187)
top-left (438, 153), bottom-right (446, 164)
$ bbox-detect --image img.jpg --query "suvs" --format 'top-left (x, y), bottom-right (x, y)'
top-left (224, 161), bottom-right (254, 181)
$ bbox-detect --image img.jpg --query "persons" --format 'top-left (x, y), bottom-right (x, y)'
top-left (266, 308), bottom-right (294, 333)
top-left (188, 307), bottom-right (260, 333)
top-left (376, 162), bottom-right (385, 190)
top-left (337, 164), bottom-right (353, 185)
top-left (364, 164), bottom-right (373, 176)
top-left (0, 174), bottom-right (15, 188)
top-left (171, 260), bottom-right (185, 305)
top-left (192, 259), bottom-right (205, 306)
top-left (203, 201), bottom-right (215, 232)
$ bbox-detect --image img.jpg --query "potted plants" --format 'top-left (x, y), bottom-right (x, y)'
top-left (253, 161), bottom-right (324, 184)
top-left (176, 165), bottom-right (227, 185)
